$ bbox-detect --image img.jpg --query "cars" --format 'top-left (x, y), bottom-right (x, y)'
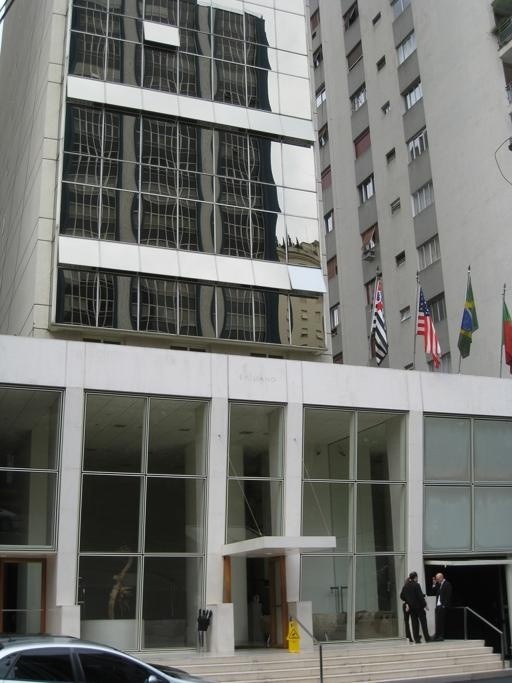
top-left (0, 633), bottom-right (208, 682)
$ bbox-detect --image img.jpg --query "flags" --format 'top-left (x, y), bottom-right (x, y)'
top-left (456, 271), bottom-right (481, 358)
top-left (502, 299), bottom-right (512, 376)
top-left (369, 279), bottom-right (389, 365)
top-left (415, 284), bottom-right (442, 369)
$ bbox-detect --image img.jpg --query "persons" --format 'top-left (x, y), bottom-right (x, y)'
top-left (255, 577), bottom-right (273, 643)
top-left (404, 571), bottom-right (435, 644)
top-left (430, 571), bottom-right (452, 642)
top-left (430, 575), bottom-right (441, 594)
top-left (400, 577), bottom-right (423, 642)
